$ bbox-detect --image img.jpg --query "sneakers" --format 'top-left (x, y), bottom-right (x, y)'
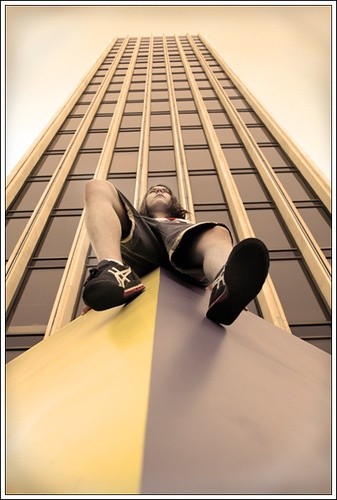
top-left (205, 233), bottom-right (269, 325)
top-left (80, 259), bottom-right (142, 307)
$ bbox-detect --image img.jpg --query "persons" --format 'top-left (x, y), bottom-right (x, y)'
top-left (81, 178), bottom-right (270, 327)
